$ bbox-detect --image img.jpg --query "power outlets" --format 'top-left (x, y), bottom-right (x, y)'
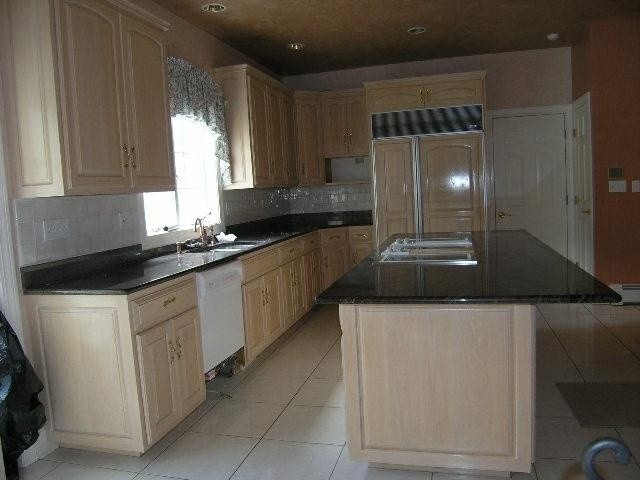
top-left (119, 211), bottom-right (130, 231)
top-left (42, 218), bottom-right (68, 241)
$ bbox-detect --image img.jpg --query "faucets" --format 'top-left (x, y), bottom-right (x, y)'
top-left (193, 217), bottom-right (209, 246)
top-left (208, 225), bottom-right (214, 240)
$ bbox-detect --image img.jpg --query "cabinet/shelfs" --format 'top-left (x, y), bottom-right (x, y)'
top-left (1, 0), bottom-right (180, 200)
top-left (126, 272), bottom-right (209, 459)
top-left (323, 89), bottom-right (367, 159)
top-left (319, 227), bottom-right (371, 298)
top-left (292, 91), bottom-right (326, 187)
top-left (241, 230), bottom-right (320, 368)
top-left (213, 68), bottom-right (294, 189)
top-left (363, 68), bottom-right (490, 248)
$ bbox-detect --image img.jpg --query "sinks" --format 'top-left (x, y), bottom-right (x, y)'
top-left (215, 241), bottom-right (263, 250)
top-left (185, 247), bottom-right (242, 252)
